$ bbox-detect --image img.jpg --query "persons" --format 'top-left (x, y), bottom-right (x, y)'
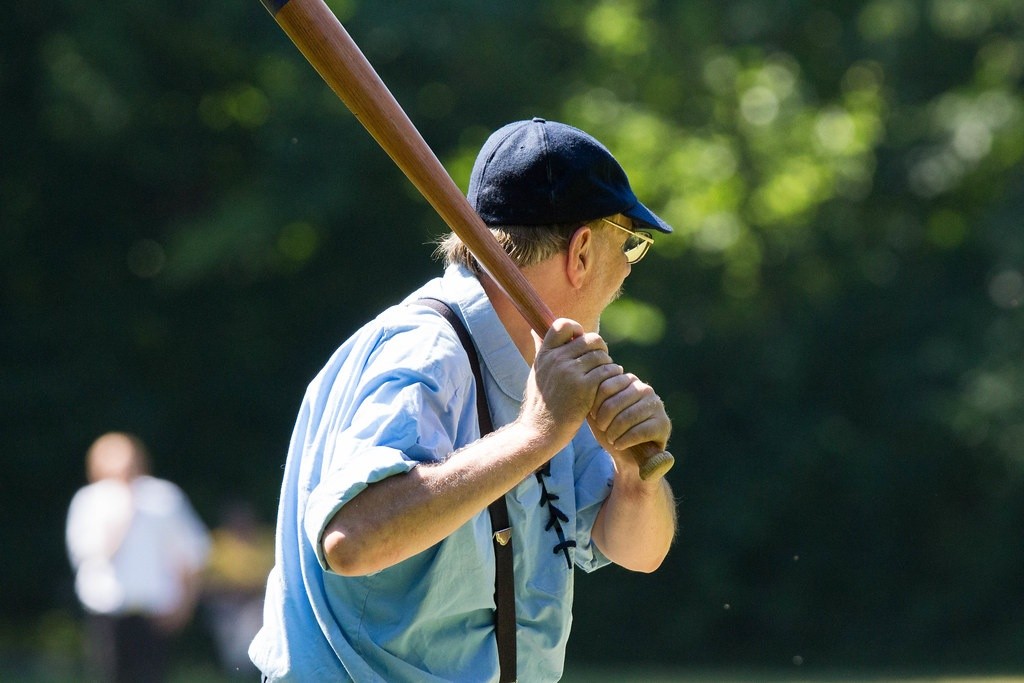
top-left (248, 117), bottom-right (677, 683)
top-left (65, 431), bottom-right (214, 683)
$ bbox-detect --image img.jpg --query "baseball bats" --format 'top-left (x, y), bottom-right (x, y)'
top-left (256, 0), bottom-right (677, 485)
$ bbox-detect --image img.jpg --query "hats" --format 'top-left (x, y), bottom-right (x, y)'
top-left (467, 117), bottom-right (674, 235)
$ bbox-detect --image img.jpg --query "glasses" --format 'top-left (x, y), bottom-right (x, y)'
top-left (601, 218), bottom-right (654, 264)
